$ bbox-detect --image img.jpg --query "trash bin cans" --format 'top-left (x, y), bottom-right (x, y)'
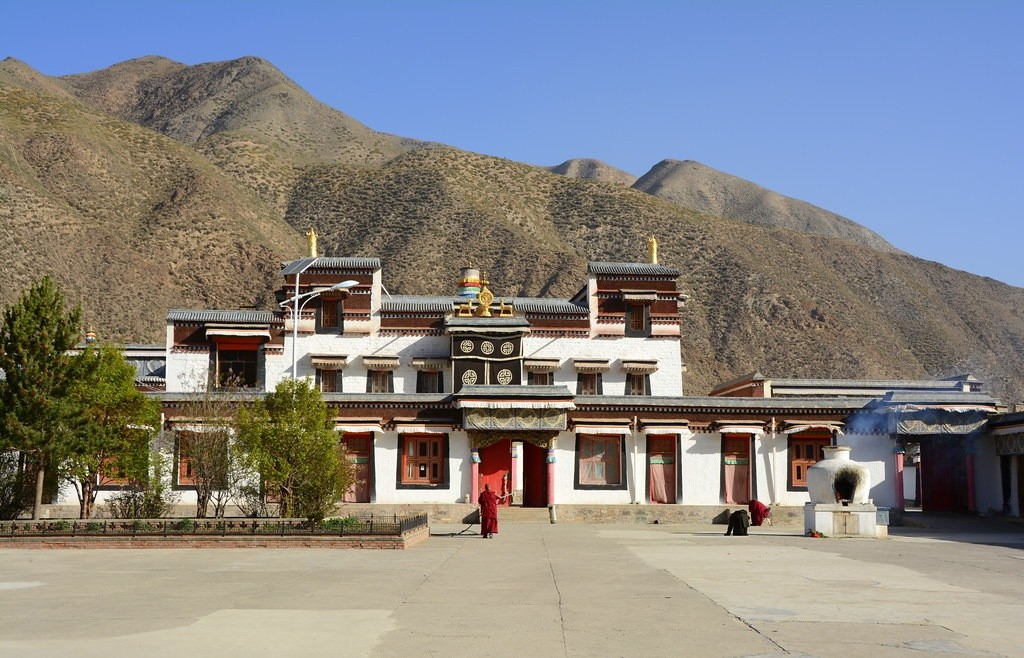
top-left (548, 504), bottom-right (557, 524)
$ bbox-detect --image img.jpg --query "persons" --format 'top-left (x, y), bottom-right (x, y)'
top-left (748, 500), bottom-right (772, 526)
top-left (724, 509), bottom-right (750, 535)
top-left (479, 484), bottom-right (502, 538)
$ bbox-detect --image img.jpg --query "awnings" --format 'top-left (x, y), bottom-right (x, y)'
top-left (205, 329), bottom-right (272, 342)
top-left (874, 404), bottom-right (998, 415)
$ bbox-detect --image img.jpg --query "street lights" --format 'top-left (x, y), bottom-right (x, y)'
top-left (278, 272), bottom-right (361, 423)
